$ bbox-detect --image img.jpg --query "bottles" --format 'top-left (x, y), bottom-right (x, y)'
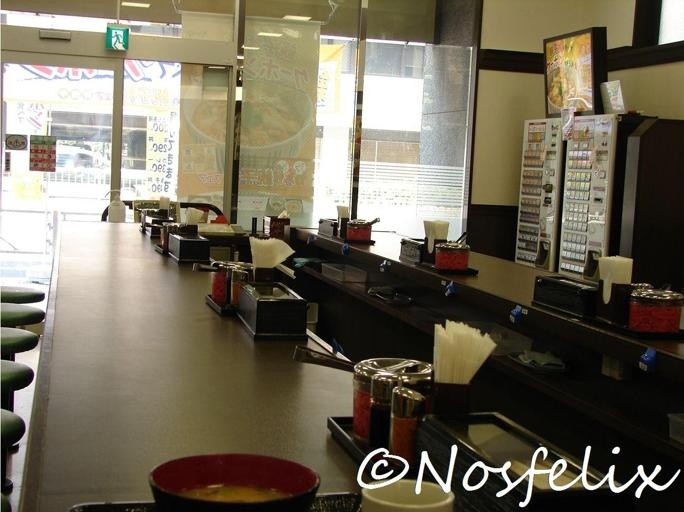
top-left (435, 241), bottom-right (470, 270)
top-left (251, 217), bottom-right (258, 234)
top-left (348, 357), bottom-right (433, 460)
top-left (106, 189), bottom-right (126, 223)
top-left (211, 259), bottom-right (252, 307)
top-left (629, 286), bottom-right (684, 335)
top-left (346, 218), bottom-right (372, 240)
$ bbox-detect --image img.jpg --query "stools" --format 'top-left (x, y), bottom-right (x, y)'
top-left (0, 285), bottom-right (45, 512)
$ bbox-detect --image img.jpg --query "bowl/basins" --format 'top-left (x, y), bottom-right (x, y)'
top-left (148, 454), bottom-right (323, 511)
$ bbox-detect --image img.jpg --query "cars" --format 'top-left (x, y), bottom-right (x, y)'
top-left (43, 144), bottom-right (150, 207)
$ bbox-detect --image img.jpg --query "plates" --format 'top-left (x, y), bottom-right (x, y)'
top-left (507, 349), bottom-right (566, 373)
top-left (375, 293), bottom-right (413, 305)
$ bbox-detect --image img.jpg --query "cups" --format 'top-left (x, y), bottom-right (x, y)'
top-left (361, 479), bottom-right (456, 511)
